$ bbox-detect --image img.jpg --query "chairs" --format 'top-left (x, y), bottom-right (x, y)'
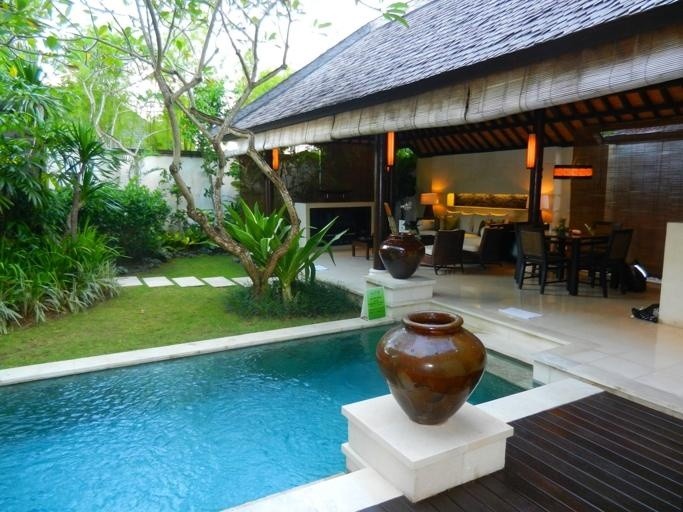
top-left (350, 229), bottom-right (372, 260)
top-left (382, 202), bottom-right (634, 298)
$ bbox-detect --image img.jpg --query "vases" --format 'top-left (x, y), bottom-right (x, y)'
top-left (379, 233), bottom-right (425, 279)
top-left (376, 311), bottom-right (486, 425)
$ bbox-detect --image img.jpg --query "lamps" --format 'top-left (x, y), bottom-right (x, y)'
top-left (420, 193), bottom-right (439, 220)
top-left (384, 132), bottom-right (395, 176)
top-left (526, 133), bottom-right (537, 169)
top-left (553, 142), bottom-right (594, 179)
top-left (447, 192), bottom-right (455, 211)
top-left (272, 148), bottom-right (279, 173)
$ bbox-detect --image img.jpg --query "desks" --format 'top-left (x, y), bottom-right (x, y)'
top-left (294, 201), bottom-right (373, 249)
